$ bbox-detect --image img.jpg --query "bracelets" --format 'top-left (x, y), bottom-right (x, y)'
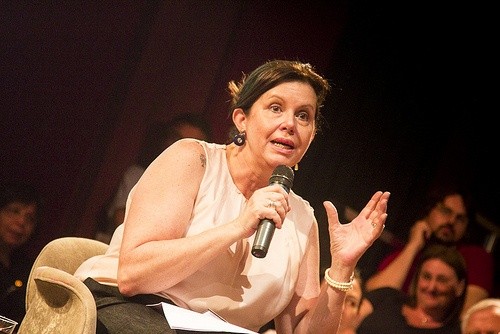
top-left (324, 269), bottom-right (354, 292)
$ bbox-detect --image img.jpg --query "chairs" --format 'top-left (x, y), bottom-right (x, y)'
top-left (25, 237), bottom-right (109, 311)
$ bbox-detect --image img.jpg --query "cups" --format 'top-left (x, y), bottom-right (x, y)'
top-left (0, 316), bottom-right (18, 334)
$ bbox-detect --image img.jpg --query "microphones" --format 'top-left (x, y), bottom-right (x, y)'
top-left (251, 165), bottom-right (294, 258)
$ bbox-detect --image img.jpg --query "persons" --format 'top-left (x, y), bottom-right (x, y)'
top-left (339, 185), bottom-right (500, 333)
top-left (74, 61), bottom-right (390, 334)
top-left (106, 116), bottom-right (210, 229)
top-left (0, 179), bottom-right (41, 334)
